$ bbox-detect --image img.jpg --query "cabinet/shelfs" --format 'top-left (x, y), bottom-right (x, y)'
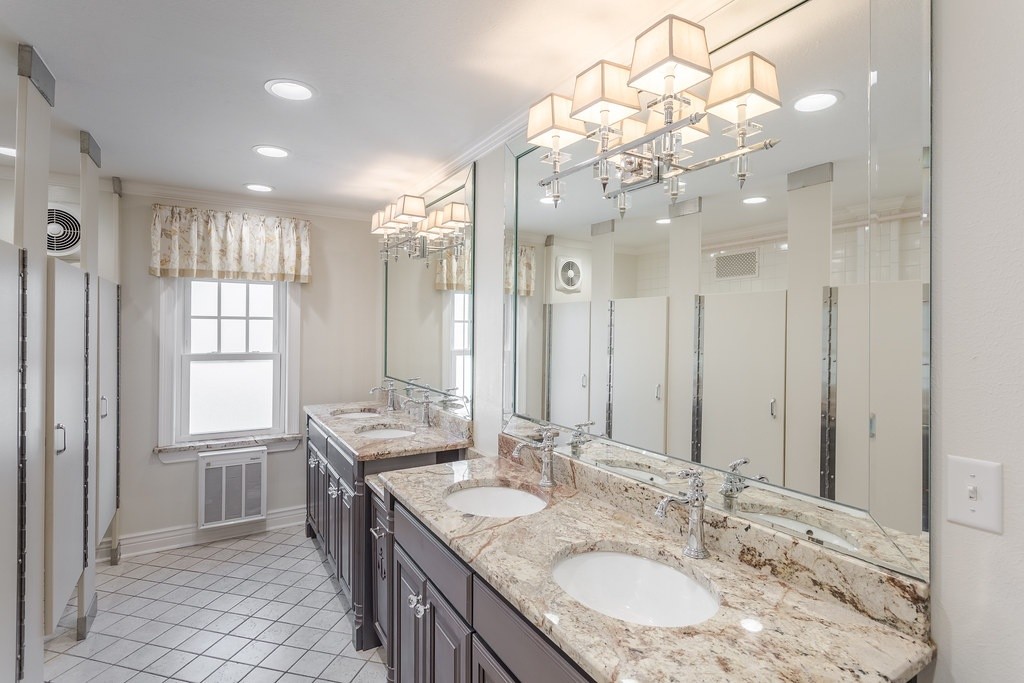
top-left (304, 415), bottom-right (595, 683)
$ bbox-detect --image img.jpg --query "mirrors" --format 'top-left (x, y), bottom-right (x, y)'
top-left (384, 160), bottom-right (477, 421)
top-left (514, 0), bottom-right (935, 535)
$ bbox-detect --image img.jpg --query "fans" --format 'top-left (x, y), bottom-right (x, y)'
top-left (46, 202), bottom-right (82, 260)
top-left (556, 255), bottom-right (583, 293)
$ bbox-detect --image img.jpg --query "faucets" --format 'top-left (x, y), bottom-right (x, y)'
top-left (655, 468), bottom-right (713, 561)
top-left (438, 386), bottom-right (465, 412)
top-left (512, 427), bottom-right (559, 490)
top-left (402, 374), bottom-right (431, 398)
top-left (718, 457), bottom-right (768, 513)
top-left (402, 383), bottom-right (434, 428)
top-left (566, 422), bottom-right (609, 459)
top-left (369, 378), bottom-right (397, 412)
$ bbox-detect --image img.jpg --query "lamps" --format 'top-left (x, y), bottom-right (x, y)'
top-left (370, 194), bottom-right (427, 265)
top-left (602, 52), bottom-right (786, 218)
top-left (528, 14), bottom-right (713, 209)
top-left (417, 202), bottom-right (471, 267)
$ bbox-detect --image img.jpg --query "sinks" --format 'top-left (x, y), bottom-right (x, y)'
top-left (353, 422), bottom-right (416, 439)
top-left (547, 537), bottom-right (721, 630)
top-left (597, 458), bottom-right (670, 488)
top-left (328, 407), bottom-right (382, 420)
top-left (441, 477), bottom-right (549, 520)
top-left (434, 402), bottom-right (464, 412)
top-left (721, 502), bottom-right (860, 556)
